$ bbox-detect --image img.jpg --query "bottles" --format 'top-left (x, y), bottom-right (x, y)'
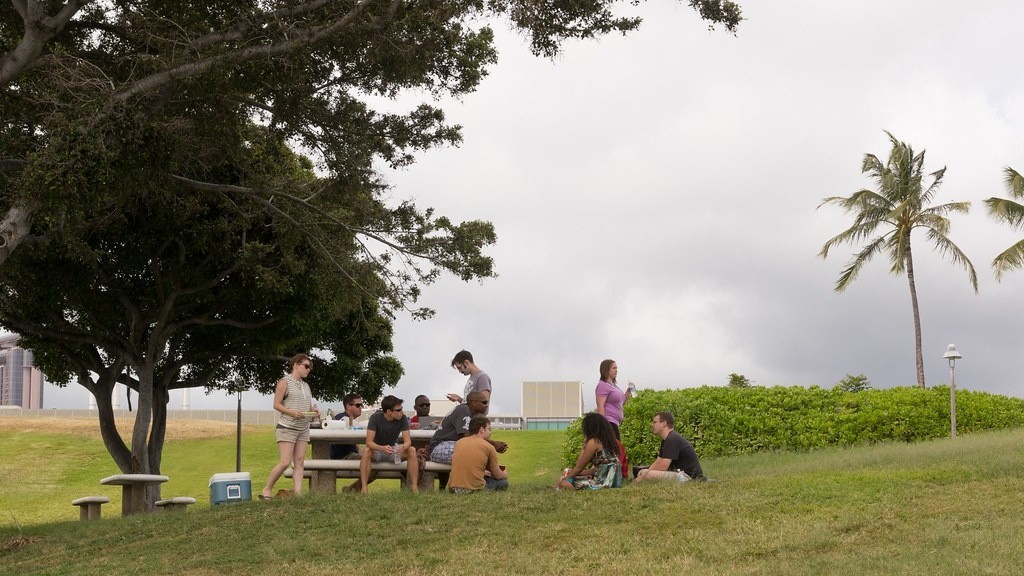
top-left (627, 380), bottom-right (638, 398)
top-left (394, 443), bottom-right (402, 464)
top-left (676, 469), bottom-right (684, 482)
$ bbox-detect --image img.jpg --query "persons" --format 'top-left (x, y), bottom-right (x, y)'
top-left (258, 354), bottom-right (319, 500)
top-left (445, 349), bottom-right (492, 415)
top-left (360, 395), bottom-right (420, 494)
top-left (448, 416), bottom-right (508, 494)
top-left (329, 394), bottom-right (377, 493)
top-left (401, 395), bottom-right (450, 491)
top-left (428, 392), bottom-right (508, 471)
top-left (548, 413), bottom-right (629, 491)
top-left (594, 359), bottom-right (638, 440)
top-left (631, 413), bottom-right (707, 482)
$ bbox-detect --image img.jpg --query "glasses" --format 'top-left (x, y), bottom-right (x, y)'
top-left (651, 420), bottom-right (665, 425)
top-left (350, 402), bottom-right (363, 408)
top-left (456, 361), bottom-right (465, 370)
top-left (469, 399), bottom-right (489, 405)
top-left (392, 407), bottom-right (404, 412)
top-left (416, 402), bottom-right (431, 408)
top-left (297, 363), bottom-right (311, 371)
top-left (485, 426), bottom-right (492, 431)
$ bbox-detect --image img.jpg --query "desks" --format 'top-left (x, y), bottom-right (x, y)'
top-left (100, 474), bottom-right (170, 486)
top-left (308, 429), bottom-right (440, 494)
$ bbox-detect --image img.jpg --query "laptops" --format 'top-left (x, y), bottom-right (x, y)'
top-left (417, 416), bottom-right (445, 431)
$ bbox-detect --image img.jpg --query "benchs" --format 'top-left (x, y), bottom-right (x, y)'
top-left (284, 458), bottom-right (454, 478)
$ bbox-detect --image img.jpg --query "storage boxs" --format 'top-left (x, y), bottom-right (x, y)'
top-left (209, 471), bottom-right (251, 507)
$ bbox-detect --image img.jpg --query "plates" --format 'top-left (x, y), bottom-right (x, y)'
top-left (301, 411), bottom-right (317, 416)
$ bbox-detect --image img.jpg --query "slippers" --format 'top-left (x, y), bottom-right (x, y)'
top-left (257, 495), bottom-right (272, 501)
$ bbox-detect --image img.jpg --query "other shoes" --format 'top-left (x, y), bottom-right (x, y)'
top-left (342, 485), bottom-right (351, 493)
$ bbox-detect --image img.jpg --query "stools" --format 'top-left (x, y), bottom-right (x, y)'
top-left (72, 496), bottom-right (109, 520)
top-left (154, 496), bottom-right (196, 511)
